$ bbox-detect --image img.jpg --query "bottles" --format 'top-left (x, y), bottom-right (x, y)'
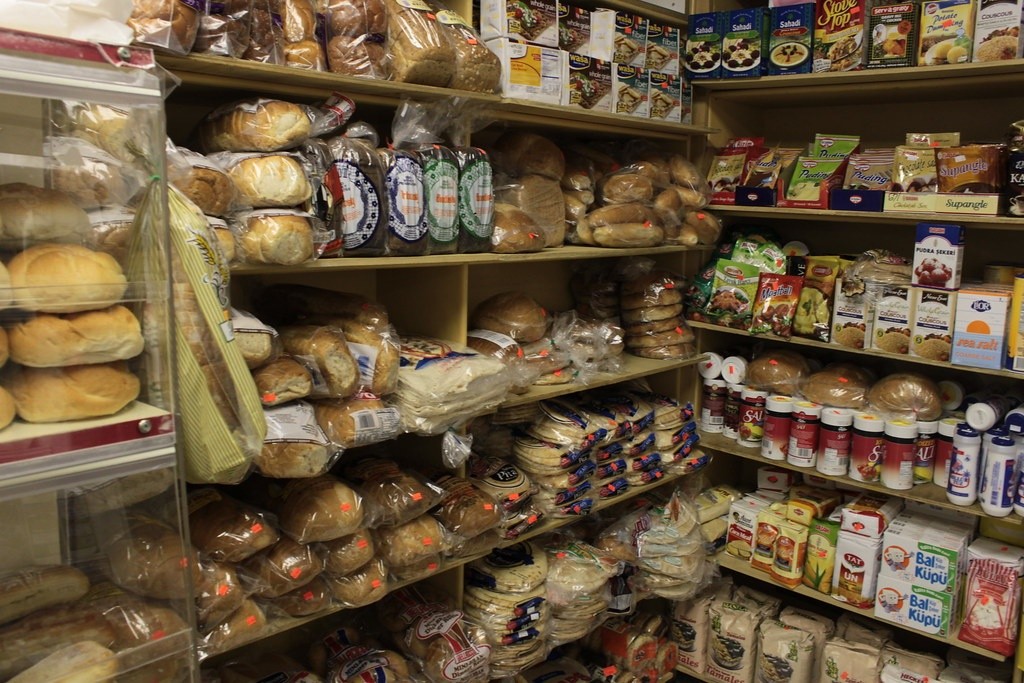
top-left (697, 348), bottom-right (1024, 517)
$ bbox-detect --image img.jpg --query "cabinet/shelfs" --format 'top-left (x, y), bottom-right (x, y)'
top-left (673, 60), bottom-right (1024, 683)
top-left (149, 51), bottom-right (721, 683)
top-left (0, 29), bottom-right (202, 683)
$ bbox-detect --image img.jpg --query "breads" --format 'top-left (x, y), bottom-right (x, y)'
top-left (747, 351), bottom-right (807, 393)
top-left (1, 0), bottom-right (719, 683)
top-left (877, 332), bottom-right (909, 352)
top-left (836, 327), bottom-right (865, 348)
top-left (802, 369), bottom-right (870, 408)
top-left (870, 372), bottom-right (943, 420)
top-left (915, 339), bottom-right (950, 359)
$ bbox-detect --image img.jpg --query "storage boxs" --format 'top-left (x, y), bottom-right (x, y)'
top-left (832, 222), bottom-right (1024, 373)
top-left (479, 0), bottom-right (1024, 126)
top-left (720, 464), bottom-right (982, 637)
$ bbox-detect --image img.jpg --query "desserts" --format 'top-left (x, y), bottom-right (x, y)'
top-left (687, 43), bottom-right (760, 69)
top-left (915, 258), bottom-right (952, 286)
